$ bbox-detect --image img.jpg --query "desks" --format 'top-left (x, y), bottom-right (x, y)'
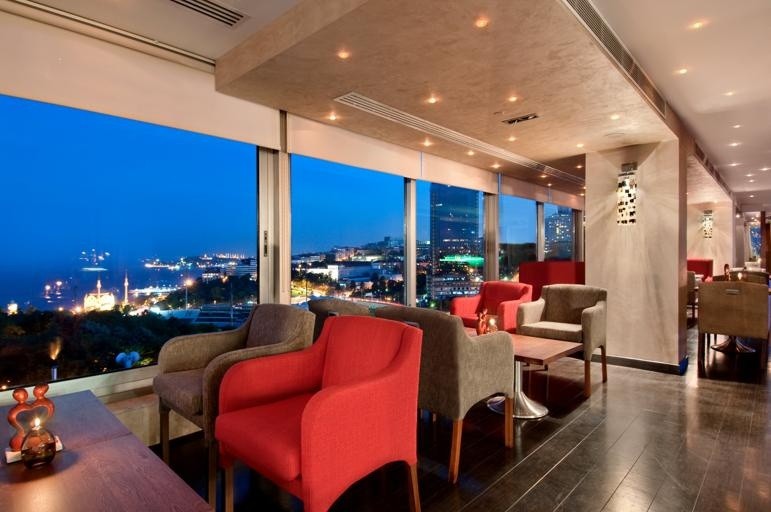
top-left (509, 332), bottom-right (584, 366)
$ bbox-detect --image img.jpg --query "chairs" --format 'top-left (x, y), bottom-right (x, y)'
top-left (375, 302), bottom-right (515, 485)
top-left (449, 279), bottom-right (532, 332)
top-left (514, 284), bottom-right (609, 395)
top-left (687, 258), bottom-right (771, 378)
top-left (307, 297), bottom-right (371, 342)
top-left (149, 303), bottom-right (318, 510)
top-left (216, 315), bottom-right (425, 512)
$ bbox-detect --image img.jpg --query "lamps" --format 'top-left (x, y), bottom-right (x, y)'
top-left (615, 171), bottom-right (637, 224)
top-left (703, 214), bottom-right (712, 238)
